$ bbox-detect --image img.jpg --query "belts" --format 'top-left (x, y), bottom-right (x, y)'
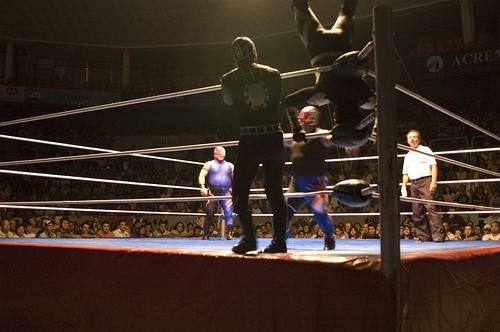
top-left (410, 175), bottom-right (432, 182)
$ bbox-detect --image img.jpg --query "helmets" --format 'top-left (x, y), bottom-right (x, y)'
top-left (299, 106), bottom-right (319, 126)
top-left (232, 37), bottom-right (257, 67)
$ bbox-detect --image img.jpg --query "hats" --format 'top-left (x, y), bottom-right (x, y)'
top-left (45, 220), bottom-right (54, 227)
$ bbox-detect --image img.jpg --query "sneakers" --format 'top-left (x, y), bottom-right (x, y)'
top-left (323, 240), bottom-right (335, 251)
top-left (259, 238), bottom-right (287, 254)
top-left (233, 239), bottom-right (256, 253)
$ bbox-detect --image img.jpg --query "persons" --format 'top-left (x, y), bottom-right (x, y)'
top-left (286, 0), bottom-right (372, 147)
top-left (0, 85), bottom-right (500, 250)
top-left (220, 37), bottom-right (288, 254)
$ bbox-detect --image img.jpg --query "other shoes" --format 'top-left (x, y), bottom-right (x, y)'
top-left (202, 235), bottom-right (209, 240)
top-left (227, 234), bottom-right (233, 240)
